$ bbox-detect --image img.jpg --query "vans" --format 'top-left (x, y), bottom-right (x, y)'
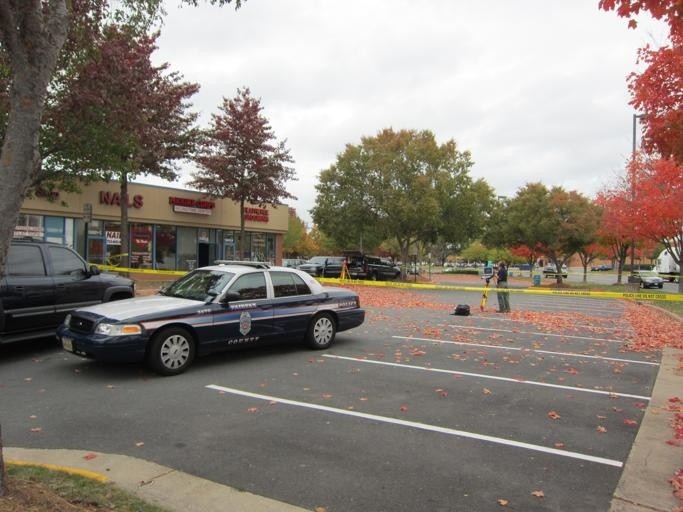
top-left (543, 265), bottom-right (567, 278)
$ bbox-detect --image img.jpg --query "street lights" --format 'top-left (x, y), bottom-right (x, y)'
top-left (630, 113), bottom-right (645, 276)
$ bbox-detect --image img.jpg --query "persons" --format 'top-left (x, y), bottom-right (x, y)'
top-left (493, 261), bottom-right (511, 314)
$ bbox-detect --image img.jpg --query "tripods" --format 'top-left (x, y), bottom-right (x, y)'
top-left (480, 282), bottom-right (498, 311)
top-left (340, 265), bottom-right (351, 280)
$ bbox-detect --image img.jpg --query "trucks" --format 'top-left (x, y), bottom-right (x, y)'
top-left (651, 247), bottom-right (681, 282)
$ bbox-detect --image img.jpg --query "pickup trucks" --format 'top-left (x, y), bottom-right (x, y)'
top-left (348, 254), bottom-right (401, 281)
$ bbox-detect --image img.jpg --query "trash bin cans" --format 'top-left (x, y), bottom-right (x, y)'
top-left (533, 275), bottom-right (540, 287)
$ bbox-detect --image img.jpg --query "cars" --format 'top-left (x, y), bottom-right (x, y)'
top-left (596, 265), bottom-right (612, 271)
top-left (407, 265), bottom-right (418, 274)
top-left (0, 236), bottom-right (136, 344)
top-left (55, 259), bottom-right (366, 375)
top-left (298, 256), bottom-right (348, 277)
top-left (628, 270), bottom-right (664, 289)
